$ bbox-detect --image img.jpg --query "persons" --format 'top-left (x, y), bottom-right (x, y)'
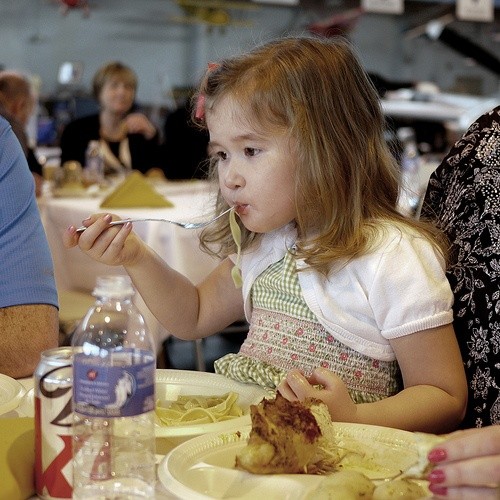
top-left (0, 115), bottom-right (59, 379)
top-left (66, 35), bottom-right (470, 433)
top-left (163, 87), bottom-right (216, 182)
top-left (58, 62), bottom-right (166, 181)
top-left (0, 72), bottom-right (46, 197)
top-left (413, 105), bottom-right (500, 500)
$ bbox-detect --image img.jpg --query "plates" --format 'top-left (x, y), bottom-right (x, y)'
top-left (158, 421), bottom-right (444, 499)
top-left (1, 373), bottom-right (27, 414)
top-left (28, 368), bottom-right (269, 457)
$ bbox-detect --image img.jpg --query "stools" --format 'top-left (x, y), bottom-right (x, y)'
top-left (58, 282), bottom-right (130, 347)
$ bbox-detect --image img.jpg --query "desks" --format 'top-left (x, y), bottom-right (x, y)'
top-left (34, 180), bottom-right (240, 366)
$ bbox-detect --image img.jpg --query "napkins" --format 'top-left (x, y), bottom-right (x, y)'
top-left (99, 174), bottom-right (174, 210)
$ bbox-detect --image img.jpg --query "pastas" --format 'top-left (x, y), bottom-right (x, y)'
top-left (154, 392), bottom-right (244, 426)
top-left (229, 206), bottom-right (243, 290)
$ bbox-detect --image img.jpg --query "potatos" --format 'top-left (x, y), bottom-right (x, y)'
top-left (302, 469), bottom-right (427, 500)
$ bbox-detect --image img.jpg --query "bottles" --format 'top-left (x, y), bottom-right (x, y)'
top-left (71, 274), bottom-right (157, 500)
top-left (86, 141), bottom-right (105, 180)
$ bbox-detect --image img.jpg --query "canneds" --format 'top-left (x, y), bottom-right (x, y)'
top-left (34, 346), bottom-right (112, 500)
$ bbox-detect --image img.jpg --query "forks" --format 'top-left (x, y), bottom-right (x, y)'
top-left (77, 205), bottom-right (237, 232)
